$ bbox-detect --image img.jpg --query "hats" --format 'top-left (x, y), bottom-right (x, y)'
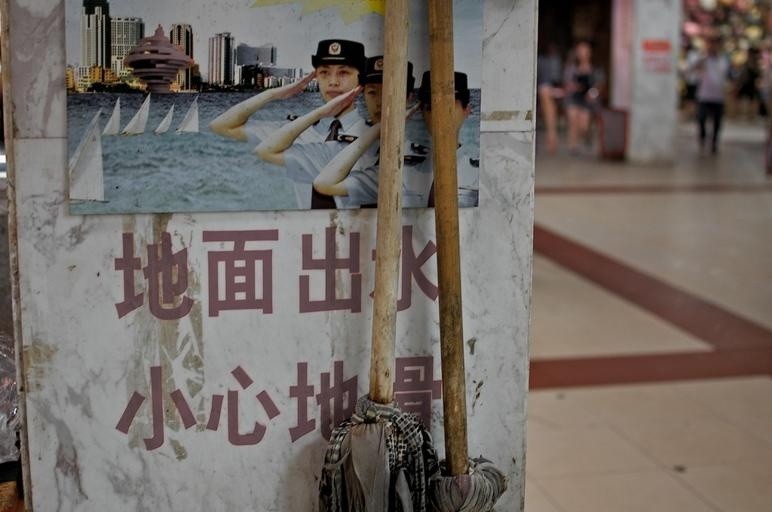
top-left (311, 40), bottom-right (364, 73)
top-left (358, 55), bottom-right (415, 93)
top-left (415, 70), bottom-right (470, 100)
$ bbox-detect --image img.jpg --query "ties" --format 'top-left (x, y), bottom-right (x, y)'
top-left (310, 119), bottom-right (342, 209)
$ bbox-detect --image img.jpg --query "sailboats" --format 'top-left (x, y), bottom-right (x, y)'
top-left (102, 92), bottom-right (199, 135)
top-left (68, 109), bottom-right (109, 204)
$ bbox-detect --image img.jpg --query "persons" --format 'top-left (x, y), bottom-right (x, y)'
top-left (254, 55), bottom-right (416, 209)
top-left (207, 39), bottom-right (375, 207)
top-left (313, 72), bottom-right (480, 211)
top-left (558, 38), bottom-right (603, 153)
top-left (679, 30), bottom-right (768, 155)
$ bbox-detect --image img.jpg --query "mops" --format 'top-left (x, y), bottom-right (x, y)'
top-left (429, 1), bottom-right (505, 512)
top-left (319, 0), bottom-right (439, 512)
